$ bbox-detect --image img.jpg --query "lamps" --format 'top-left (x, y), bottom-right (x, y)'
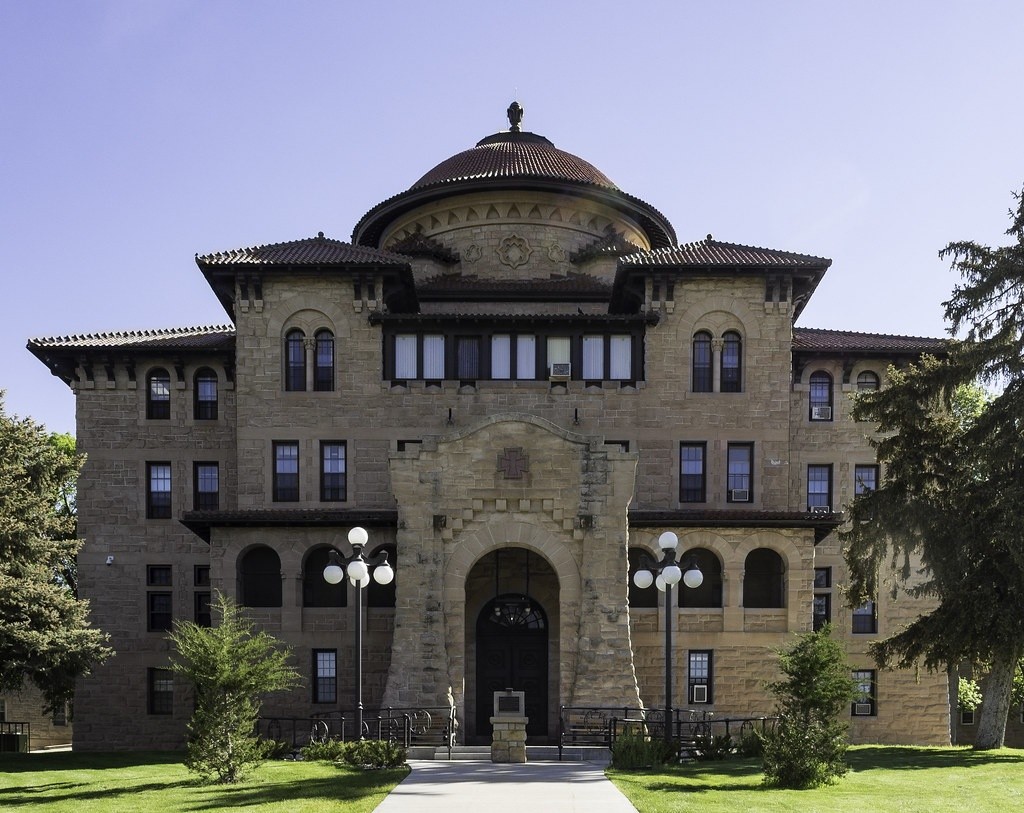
top-left (106, 555), bottom-right (114, 566)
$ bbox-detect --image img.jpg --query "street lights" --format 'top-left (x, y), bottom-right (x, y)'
top-left (632, 532), bottom-right (704, 763)
top-left (323, 526), bottom-right (394, 739)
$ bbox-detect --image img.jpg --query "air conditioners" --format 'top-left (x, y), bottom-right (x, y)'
top-left (548, 362), bottom-right (571, 378)
top-left (855, 704), bottom-right (871, 715)
top-left (812, 406), bottom-right (832, 419)
top-left (694, 685), bottom-right (707, 702)
top-left (732, 489), bottom-right (750, 501)
top-left (810, 506), bottom-right (830, 513)
